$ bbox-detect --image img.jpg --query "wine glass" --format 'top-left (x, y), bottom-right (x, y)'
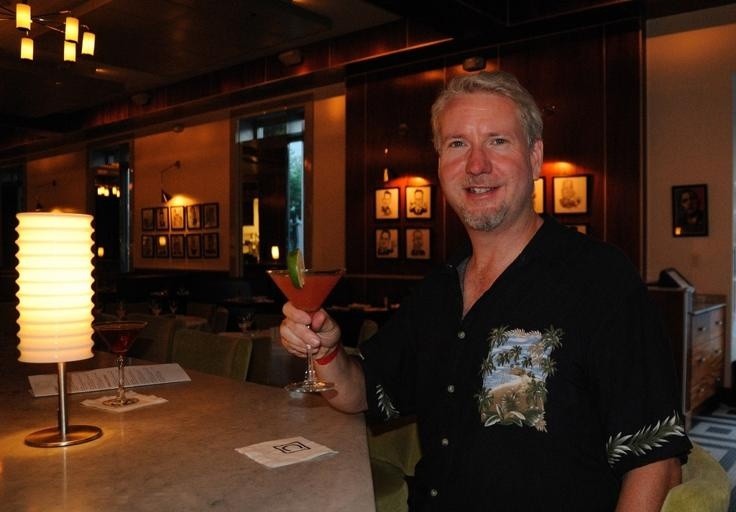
top-left (238, 312), bottom-right (254, 339)
top-left (153, 304), bottom-right (160, 315)
top-left (169, 301), bottom-right (178, 315)
top-left (267, 269), bottom-right (345, 394)
top-left (94, 320), bottom-right (149, 407)
top-left (114, 299), bottom-right (128, 320)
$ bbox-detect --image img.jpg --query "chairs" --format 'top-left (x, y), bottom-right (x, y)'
top-left (186, 303), bottom-right (217, 333)
top-left (367, 416), bottom-right (422, 512)
top-left (252, 337), bottom-right (273, 376)
top-left (153, 278), bottom-right (183, 308)
top-left (120, 316), bottom-right (173, 363)
top-left (658, 443), bottom-right (731, 511)
top-left (172, 328), bottom-right (252, 382)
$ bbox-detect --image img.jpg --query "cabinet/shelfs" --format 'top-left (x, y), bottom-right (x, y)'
top-left (647, 285), bottom-right (726, 431)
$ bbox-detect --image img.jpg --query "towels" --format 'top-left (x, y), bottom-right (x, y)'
top-left (234, 436), bottom-right (336, 468)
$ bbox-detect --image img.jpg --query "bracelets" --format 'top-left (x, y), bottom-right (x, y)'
top-left (313, 342), bottom-right (342, 366)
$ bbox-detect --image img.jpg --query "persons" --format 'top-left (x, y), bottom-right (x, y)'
top-left (380, 191), bottom-right (393, 217)
top-left (678, 189), bottom-right (705, 228)
top-left (409, 190), bottom-right (426, 215)
top-left (277, 70), bottom-right (694, 510)
top-left (378, 230), bottom-right (393, 256)
top-left (411, 230), bottom-right (425, 257)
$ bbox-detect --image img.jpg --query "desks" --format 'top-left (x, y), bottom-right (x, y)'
top-left (102, 312), bottom-right (207, 333)
top-left (220, 328), bottom-right (283, 345)
top-left (107, 270), bottom-right (183, 297)
top-left (0, 345), bottom-right (376, 511)
top-left (250, 312), bottom-right (284, 325)
top-left (223, 296), bottom-right (275, 314)
top-left (199, 280), bottom-right (250, 295)
top-left (325, 303), bottom-right (394, 323)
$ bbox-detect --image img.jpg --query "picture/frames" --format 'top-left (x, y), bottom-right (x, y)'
top-left (170, 206), bottom-right (184, 229)
top-left (406, 185), bottom-right (432, 220)
top-left (203, 204), bottom-right (217, 229)
top-left (673, 184), bottom-right (708, 236)
top-left (532, 176), bottom-right (545, 214)
top-left (203, 233), bottom-right (217, 257)
top-left (405, 227), bottom-right (432, 260)
top-left (141, 208), bottom-right (154, 230)
top-left (373, 187), bottom-right (399, 221)
top-left (171, 234), bottom-right (183, 258)
top-left (155, 208), bottom-right (168, 230)
top-left (187, 234), bottom-right (200, 258)
top-left (552, 175), bottom-right (590, 214)
top-left (374, 228), bottom-right (399, 259)
top-left (187, 205), bottom-right (201, 229)
top-left (141, 235), bottom-right (154, 258)
top-left (154, 234), bottom-right (168, 258)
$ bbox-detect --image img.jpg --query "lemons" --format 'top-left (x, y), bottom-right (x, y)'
top-left (286, 248), bottom-right (305, 289)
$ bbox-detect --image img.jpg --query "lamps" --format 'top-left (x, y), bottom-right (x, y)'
top-left (1, 0), bottom-right (96, 64)
top-left (160, 160), bottom-right (180, 203)
top-left (95, 180), bottom-right (121, 203)
top-left (14, 211), bottom-right (102, 448)
